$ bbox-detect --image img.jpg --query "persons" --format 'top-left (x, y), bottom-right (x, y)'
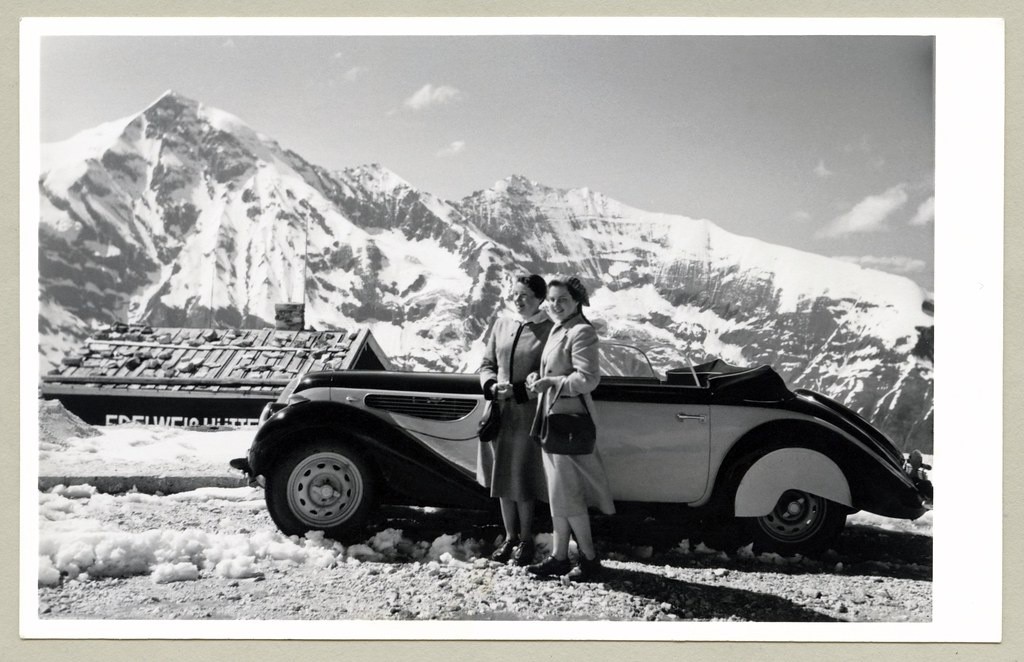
top-left (525, 279), bottom-right (616, 583)
top-left (476, 274), bottom-right (554, 565)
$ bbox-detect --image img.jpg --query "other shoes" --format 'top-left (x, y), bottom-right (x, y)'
top-left (525, 555), bottom-right (571, 576)
top-left (565, 550), bottom-right (602, 582)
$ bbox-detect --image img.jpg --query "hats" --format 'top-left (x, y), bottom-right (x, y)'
top-left (550, 276), bottom-right (590, 307)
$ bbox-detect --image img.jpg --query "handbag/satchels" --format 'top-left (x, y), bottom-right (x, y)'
top-left (540, 378), bottom-right (596, 455)
top-left (478, 399), bottom-right (502, 442)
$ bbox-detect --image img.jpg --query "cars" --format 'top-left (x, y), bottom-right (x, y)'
top-left (229, 339), bottom-right (933, 569)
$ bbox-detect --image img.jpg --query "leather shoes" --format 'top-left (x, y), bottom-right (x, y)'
top-left (492, 536), bottom-right (520, 561)
top-left (513, 537), bottom-right (534, 565)
